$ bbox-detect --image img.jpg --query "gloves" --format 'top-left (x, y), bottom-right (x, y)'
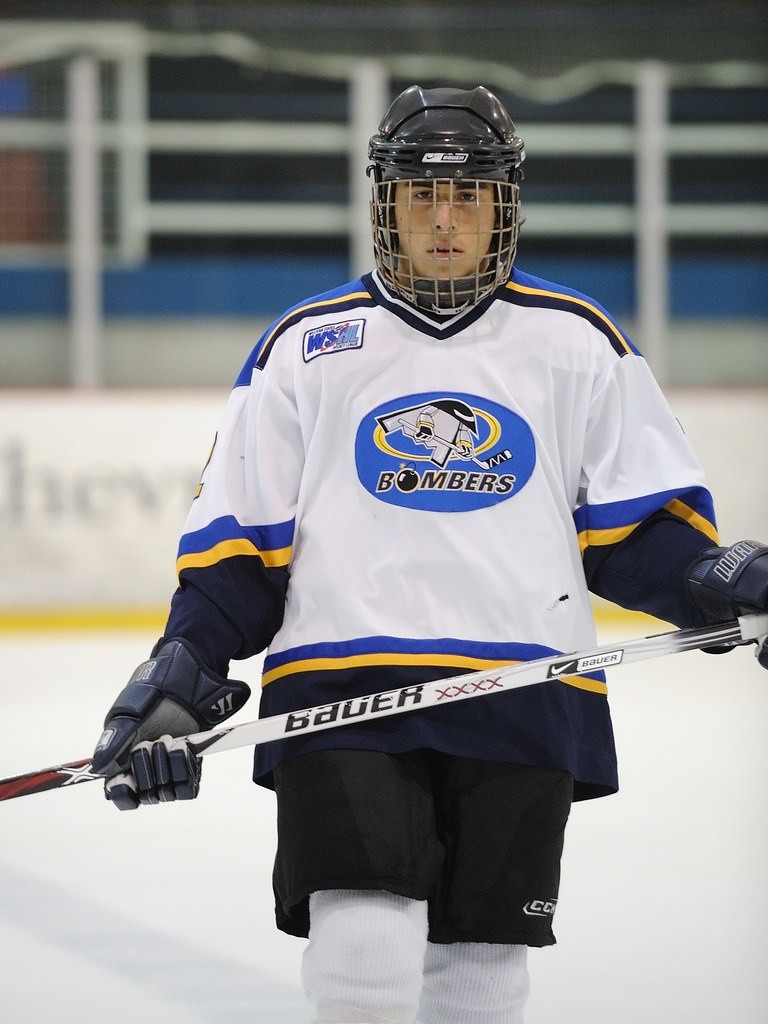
top-left (685, 541), bottom-right (767, 670)
top-left (93, 638), bottom-right (251, 812)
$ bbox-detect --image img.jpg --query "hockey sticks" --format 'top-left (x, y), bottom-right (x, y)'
top-left (1, 608), bottom-right (768, 802)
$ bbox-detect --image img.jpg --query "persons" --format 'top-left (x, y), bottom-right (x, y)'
top-left (92, 84), bottom-right (768, 1024)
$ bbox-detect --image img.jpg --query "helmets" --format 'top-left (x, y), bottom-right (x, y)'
top-left (368, 85), bottom-right (526, 253)
